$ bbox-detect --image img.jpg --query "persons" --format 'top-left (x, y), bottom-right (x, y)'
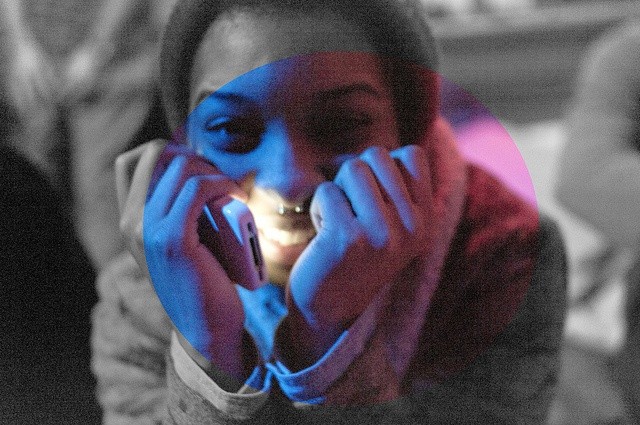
top-left (85, 1), bottom-right (565, 424)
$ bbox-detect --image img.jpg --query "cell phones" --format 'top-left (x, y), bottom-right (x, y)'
top-left (199, 196), bottom-right (269, 290)
